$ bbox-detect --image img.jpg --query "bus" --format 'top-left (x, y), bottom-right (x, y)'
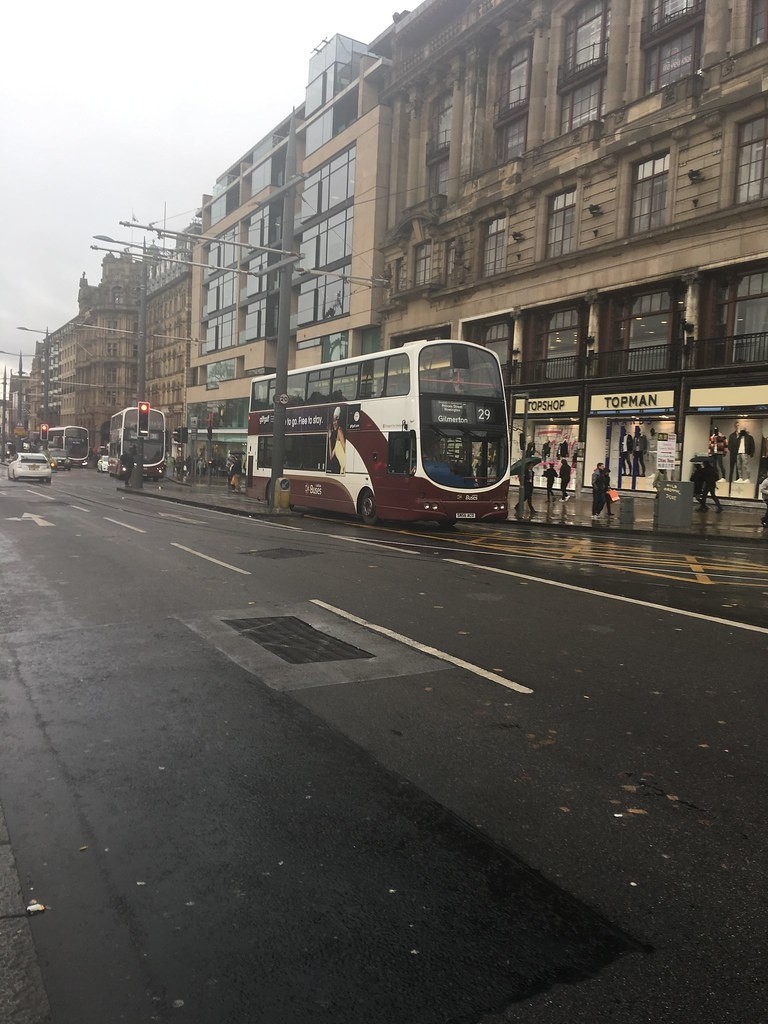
top-left (47, 425), bottom-right (90, 467)
top-left (247, 337), bottom-right (526, 527)
top-left (108, 407), bottom-right (171, 481)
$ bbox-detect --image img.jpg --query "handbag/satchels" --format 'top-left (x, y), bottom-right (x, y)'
top-left (606, 488), bottom-right (620, 503)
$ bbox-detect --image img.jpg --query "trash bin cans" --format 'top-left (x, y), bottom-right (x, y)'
top-left (653, 481), bottom-right (694, 528)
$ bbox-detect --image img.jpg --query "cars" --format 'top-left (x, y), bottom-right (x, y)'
top-left (96, 455), bottom-right (109, 473)
top-left (49, 448), bottom-right (72, 471)
top-left (7, 452), bottom-right (51, 483)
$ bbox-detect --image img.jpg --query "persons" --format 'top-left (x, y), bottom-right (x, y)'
top-left (633, 427), bottom-right (647, 477)
top-left (88, 446), bottom-right (247, 495)
top-left (619, 429), bottom-right (633, 476)
top-left (653, 469), bottom-right (667, 499)
top-left (708, 422), bottom-right (755, 483)
top-left (514, 459), bottom-right (614, 518)
top-left (697, 461), bottom-right (724, 514)
top-left (760, 477), bottom-right (768, 527)
top-left (328, 407), bottom-right (345, 475)
top-left (690, 464), bottom-right (710, 512)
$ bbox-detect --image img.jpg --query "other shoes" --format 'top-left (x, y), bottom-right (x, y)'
top-left (703, 506), bottom-right (710, 511)
top-left (607, 512), bottom-right (614, 515)
top-left (592, 514), bottom-right (601, 520)
top-left (716, 508), bottom-right (723, 513)
top-left (717, 478), bottom-right (727, 483)
top-left (559, 498), bottom-right (565, 502)
top-left (552, 496), bottom-right (557, 502)
top-left (741, 479), bottom-right (750, 483)
top-left (734, 478), bottom-right (743, 483)
top-left (565, 496), bottom-right (570, 501)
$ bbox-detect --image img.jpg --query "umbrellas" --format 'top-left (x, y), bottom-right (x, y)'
top-left (690, 456), bottom-right (715, 462)
top-left (230, 450), bottom-right (245, 456)
top-left (510, 457), bottom-right (541, 475)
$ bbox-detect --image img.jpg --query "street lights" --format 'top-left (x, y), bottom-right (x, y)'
top-left (17, 327), bottom-right (50, 424)
top-left (0, 351), bottom-right (22, 452)
top-left (94, 234), bottom-right (147, 400)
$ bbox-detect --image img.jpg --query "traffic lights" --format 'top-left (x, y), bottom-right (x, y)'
top-left (136, 401), bottom-right (149, 438)
top-left (40, 424), bottom-right (49, 441)
